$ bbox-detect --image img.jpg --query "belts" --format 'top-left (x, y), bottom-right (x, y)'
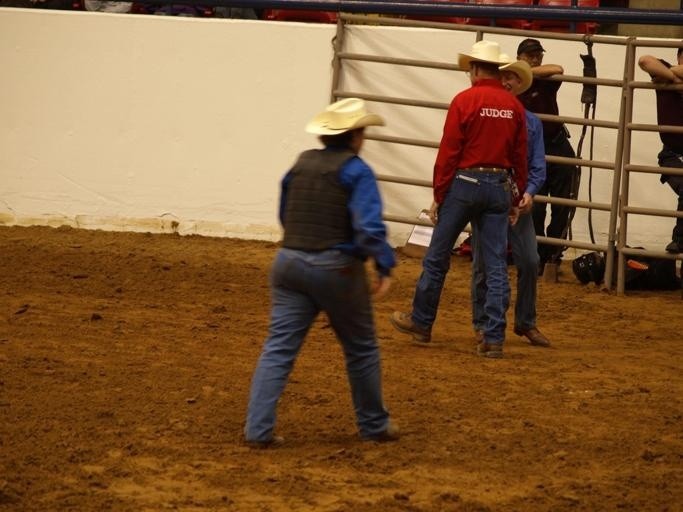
top-left (458, 167), bottom-right (510, 174)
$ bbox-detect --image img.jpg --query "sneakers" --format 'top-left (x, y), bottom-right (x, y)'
top-left (665, 240), bottom-right (683, 252)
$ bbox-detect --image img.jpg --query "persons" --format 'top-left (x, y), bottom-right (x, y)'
top-left (240, 97), bottom-right (401, 445)
top-left (391, 39), bottom-right (530, 358)
top-left (637, 45), bottom-right (682, 255)
top-left (469, 50), bottom-right (551, 348)
top-left (515, 38), bottom-right (577, 275)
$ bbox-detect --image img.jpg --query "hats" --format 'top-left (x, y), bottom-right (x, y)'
top-left (517, 38), bottom-right (546, 56)
top-left (304, 98), bottom-right (385, 136)
top-left (498, 59), bottom-right (534, 97)
top-left (457, 40), bottom-right (512, 72)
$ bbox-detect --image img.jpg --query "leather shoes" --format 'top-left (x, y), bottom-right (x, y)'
top-left (388, 310), bottom-right (432, 347)
top-left (358, 424), bottom-right (401, 442)
top-left (476, 341), bottom-right (504, 359)
top-left (244, 435), bottom-right (285, 449)
top-left (513, 323), bottom-right (550, 347)
